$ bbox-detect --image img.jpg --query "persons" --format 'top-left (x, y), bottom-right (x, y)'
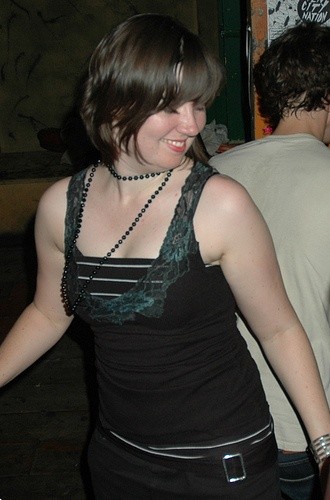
top-left (0, 14), bottom-right (330, 500)
top-left (205, 23), bottom-right (330, 499)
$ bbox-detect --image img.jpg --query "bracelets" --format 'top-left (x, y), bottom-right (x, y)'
top-left (306, 433), bottom-right (330, 465)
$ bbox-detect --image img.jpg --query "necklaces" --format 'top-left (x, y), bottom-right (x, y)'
top-left (62, 159), bottom-right (173, 317)
